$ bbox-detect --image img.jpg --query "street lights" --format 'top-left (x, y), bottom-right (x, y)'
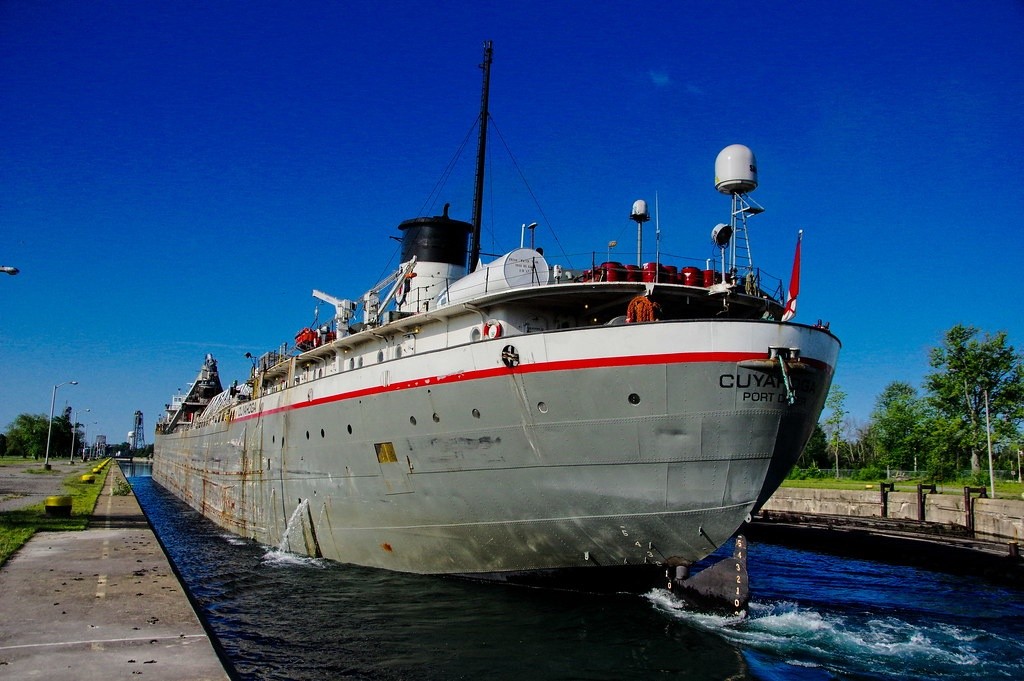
top-left (85, 421), bottom-right (97, 459)
top-left (42, 381), bottom-right (78, 470)
top-left (68, 409), bottom-right (90, 466)
top-left (980, 380), bottom-right (995, 499)
top-left (836, 411), bottom-right (849, 478)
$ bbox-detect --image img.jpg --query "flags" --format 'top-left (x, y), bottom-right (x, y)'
top-left (781, 236), bottom-right (801, 322)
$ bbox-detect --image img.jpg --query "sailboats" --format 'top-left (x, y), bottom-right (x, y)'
top-left (150, 41), bottom-right (843, 624)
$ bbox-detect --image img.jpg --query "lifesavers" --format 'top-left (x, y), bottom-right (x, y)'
top-left (485, 319), bottom-right (503, 340)
